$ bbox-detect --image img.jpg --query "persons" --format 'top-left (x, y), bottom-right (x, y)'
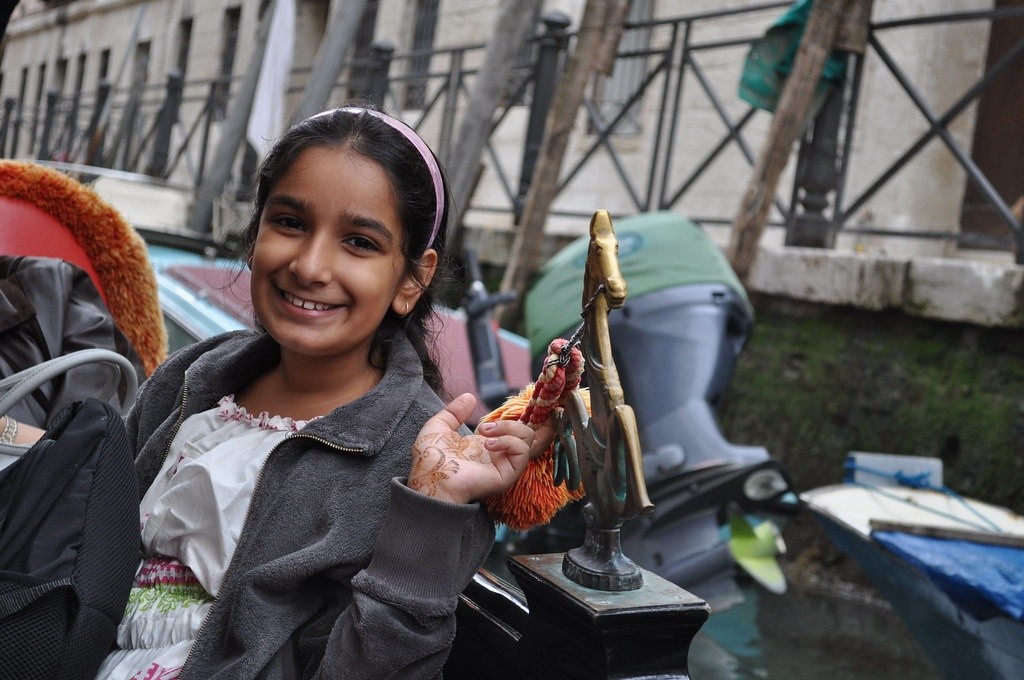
top-left (93, 104), bottom-right (570, 679)
